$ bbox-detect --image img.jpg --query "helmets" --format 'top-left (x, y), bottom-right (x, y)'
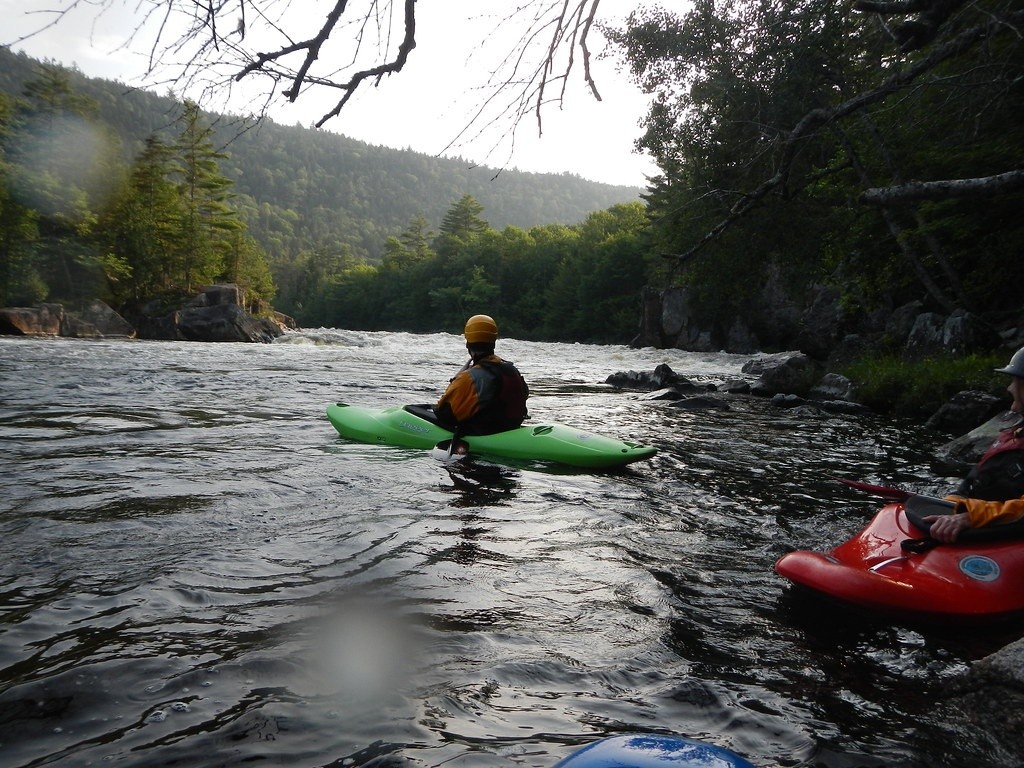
top-left (994, 346), bottom-right (1024, 378)
top-left (465, 315), bottom-right (498, 343)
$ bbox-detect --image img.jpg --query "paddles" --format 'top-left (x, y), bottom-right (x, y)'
top-left (813, 470), bottom-right (960, 506)
top-left (432, 358), bottom-right (474, 462)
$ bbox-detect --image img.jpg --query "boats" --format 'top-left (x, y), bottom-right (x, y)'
top-left (326, 403), bottom-right (658, 467)
top-left (773, 495), bottom-right (1024, 624)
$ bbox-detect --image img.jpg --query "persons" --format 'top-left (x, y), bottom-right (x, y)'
top-left (921, 345), bottom-right (1024, 545)
top-left (432, 313), bottom-right (530, 436)
